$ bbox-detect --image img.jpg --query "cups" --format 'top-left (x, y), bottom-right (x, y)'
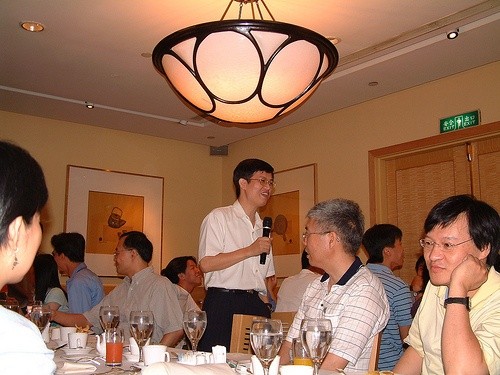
top-left (0, 299), bottom-right (88, 348)
top-left (129, 336), bottom-right (151, 355)
top-left (251, 338), bottom-right (314, 375)
top-left (212, 345), bottom-right (227, 364)
top-left (106, 329), bottom-right (124, 366)
top-left (142, 345), bottom-right (171, 366)
top-left (99, 305), bottom-right (120, 331)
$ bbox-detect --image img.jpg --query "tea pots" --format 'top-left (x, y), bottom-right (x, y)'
top-left (96, 329), bottom-right (106, 356)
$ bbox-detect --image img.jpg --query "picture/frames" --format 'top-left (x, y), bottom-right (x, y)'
top-left (59, 164), bottom-right (164, 279)
top-left (256, 162), bottom-right (318, 279)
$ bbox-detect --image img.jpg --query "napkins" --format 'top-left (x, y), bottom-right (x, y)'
top-left (54, 361), bottom-right (97, 375)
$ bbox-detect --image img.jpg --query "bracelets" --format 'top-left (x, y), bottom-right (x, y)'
top-left (264, 303), bottom-right (273, 309)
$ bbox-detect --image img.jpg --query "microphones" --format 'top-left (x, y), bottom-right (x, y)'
top-left (260, 217), bottom-right (273, 265)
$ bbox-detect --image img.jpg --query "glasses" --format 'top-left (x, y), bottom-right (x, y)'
top-left (245, 178), bottom-right (277, 189)
top-left (420, 238), bottom-right (472, 251)
top-left (113, 250), bottom-right (138, 255)
top-left (302, 232), bottom-right (340, 242)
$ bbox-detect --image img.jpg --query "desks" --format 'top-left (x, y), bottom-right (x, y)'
top-left (37, 327), bottom-right (403, 375)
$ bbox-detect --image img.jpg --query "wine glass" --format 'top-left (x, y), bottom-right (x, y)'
top-left (300, 318), bottom-right (333, 375)
top-left (183, 311), bottom-right (207, 353)
top-left (250, 319), bottom-right (283, 375)
top-left (129, 311), bottom-right (154, 362)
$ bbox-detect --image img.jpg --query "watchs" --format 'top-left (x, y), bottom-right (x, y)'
top-left (413, 290), bottom-right (425, 297)
top-left (444, 296), bottom-right (472, 312)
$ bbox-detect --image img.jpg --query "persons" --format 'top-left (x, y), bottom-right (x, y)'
top-left (0, 139), bottom-right (202, 375)
top-left (391, 193), bottom-right (500, 375)
top-left (264, 195), bottom-right (430, 375)
top-left (196, 156), bottom-right (275, 353)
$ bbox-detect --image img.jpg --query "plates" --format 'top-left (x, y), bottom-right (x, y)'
top-left (122, 352), bottom-right (141, 362)
top-left (133, 362), bottom-right (146, 370)
top-left (57, 341), bottom-right (95, 355)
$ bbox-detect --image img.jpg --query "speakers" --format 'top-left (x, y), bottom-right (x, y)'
top-left (210, 146), bottom-right (229, 155)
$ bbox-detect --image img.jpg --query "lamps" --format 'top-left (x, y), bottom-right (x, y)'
top-left (152, 0), bottom-right (339, 124)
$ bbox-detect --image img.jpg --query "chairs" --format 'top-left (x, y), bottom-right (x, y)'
top-left (230, 314), bottom-right (268, 356)
top-left (368, 329), bottom-right (383, 372)
top-left (271, 311), bottom-right (298, 359)
